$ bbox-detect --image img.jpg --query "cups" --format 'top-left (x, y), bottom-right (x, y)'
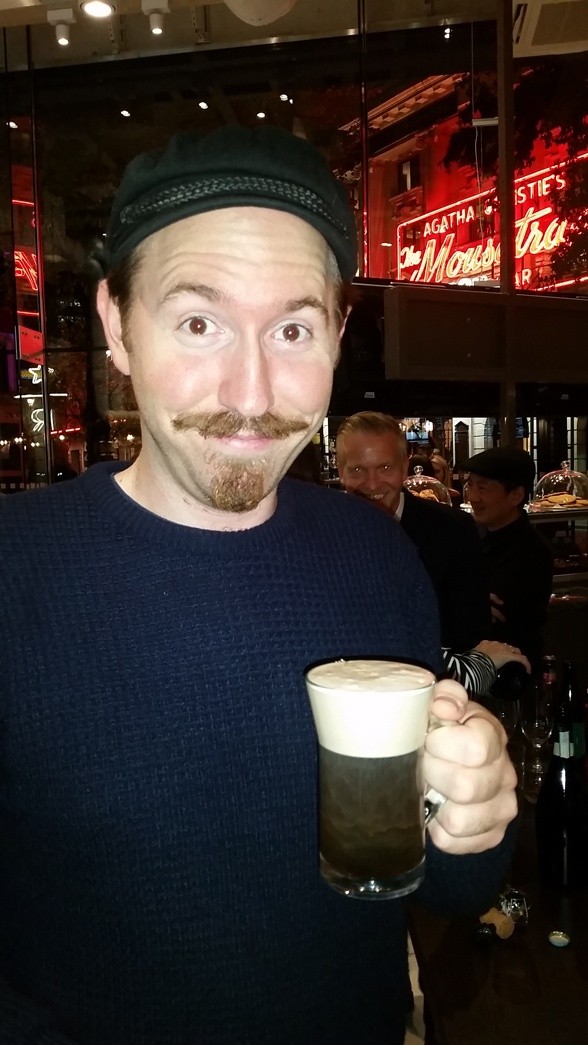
top-left (491, 689), bottom-right (551, 807)
top-left (306, 656), bottom-right (457, 900)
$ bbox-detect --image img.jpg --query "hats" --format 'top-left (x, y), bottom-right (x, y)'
top-left (408, 454), bottom-right (435, 477)
top-left (104, 124), bottom-right (359, 285)
top-left (460, 448), bottom-right (536, 488)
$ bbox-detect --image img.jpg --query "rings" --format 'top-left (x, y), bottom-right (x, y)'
top-left (455, 700), bottom-right (510, 757)
top-left (509, 645), bottom-right (515, 654)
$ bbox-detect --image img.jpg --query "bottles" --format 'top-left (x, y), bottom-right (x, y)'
top-left (536, 652), bottom-right (587, 898)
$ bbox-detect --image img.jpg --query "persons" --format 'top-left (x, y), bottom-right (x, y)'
top-left (437, 639), bottom-right (531, 703)
top-left (332, 407), bottom-right (527, 1044)
top-left (451, 443), bottom-right (555, 957)
top-left (0, 120), bottom-right (521, 1045)
